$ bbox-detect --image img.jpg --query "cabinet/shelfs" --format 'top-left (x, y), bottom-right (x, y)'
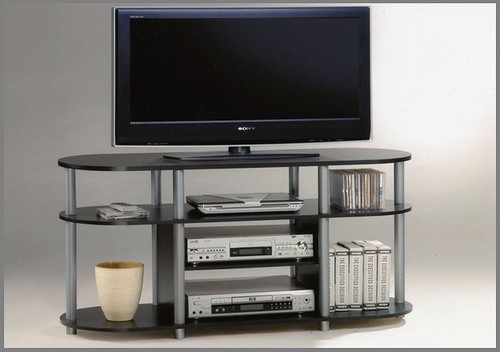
top-left (57, 147), bottom-right (413, 339)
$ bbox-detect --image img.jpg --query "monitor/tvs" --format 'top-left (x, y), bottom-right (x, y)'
top-left (110, 4), bottom-right (373, 147)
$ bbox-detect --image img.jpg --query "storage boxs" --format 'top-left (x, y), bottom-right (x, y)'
top-left (93, 261), bottom-right (145, 323)
